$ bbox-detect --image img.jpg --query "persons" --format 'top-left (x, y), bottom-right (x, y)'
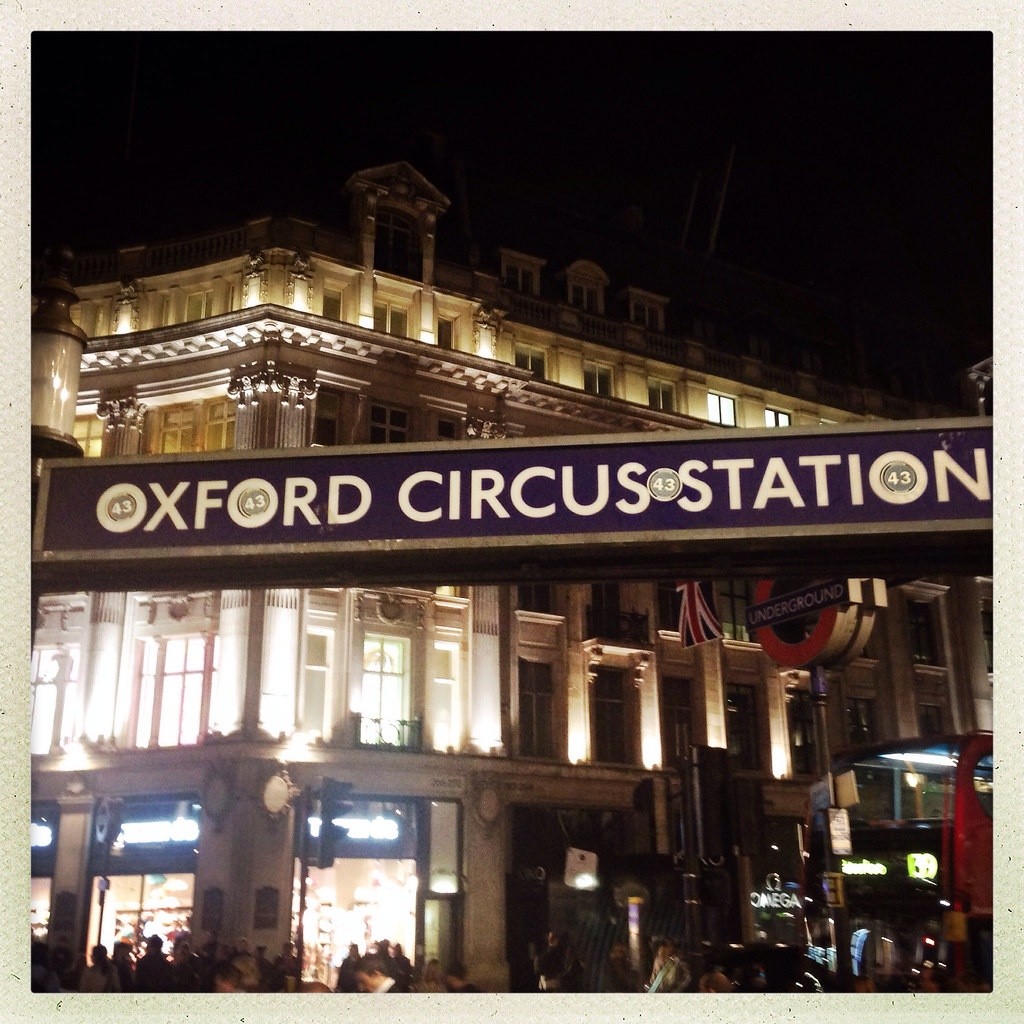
top-left (518, 928), bottom-right (995, 993)
top-left (31, 933), bottom-right (485, 993)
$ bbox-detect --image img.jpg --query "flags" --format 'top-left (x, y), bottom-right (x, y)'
top-left (674, 577), bottom-right (724, 650)
